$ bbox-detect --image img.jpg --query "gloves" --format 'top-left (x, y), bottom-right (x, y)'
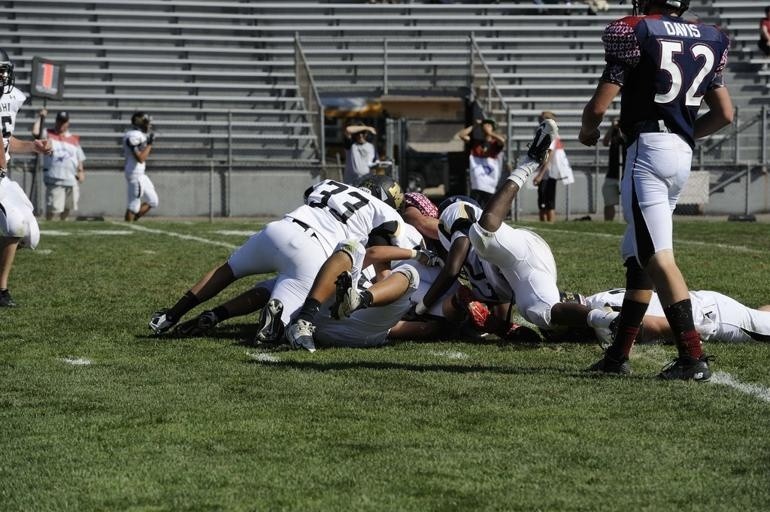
top-left (421, 248), bottom-right (440, 268)
top-left (145, 132), bottom-right (155, 145)
top-left (400, 304), bottom-right (420, 323)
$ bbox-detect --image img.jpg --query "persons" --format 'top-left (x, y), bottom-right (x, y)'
top-left (343, 117), bottom-right (381, 185)
top-left (32, 109), bottom-right (87, 221)
top-left (147, 174), bottom-right (769, 353)
top-left (534, 111), bottom-right (575, 221)
top-left (0, 48), bottom-right (41, 307)
top-left (123, 110), bottom-right (159, 223)
top-left (757, 6), bottom-right (770, 56)
top-left (460, 117), bottom-right (505, 207)
top-left (578, 0), bottom-right (736, 384)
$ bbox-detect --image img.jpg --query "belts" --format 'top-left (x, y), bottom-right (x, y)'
top-left (285, 213), bottom-right (320, 243)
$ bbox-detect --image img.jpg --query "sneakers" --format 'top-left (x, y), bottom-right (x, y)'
top-left (172, 308), bottom-right (219, 340)
top-left (609, 312), bottom-right (646, 345)
top-left (526, 118), bottom-right (559, 168)
top-left (656, 355), bottom-right (714, 382)
top-left (327, 269), bottom-right (363, 323)
top-left (284, 317), bottom-right (320, 356)
top-left (456, 285), bottom-right (498, 335)
top-left (573, 348), bottom-right (633, 378)
top-left (147, 309), bottom-right (181, 334)
top-left (504, 324), bottom-right (542, 345)
top-left (0, 289), bottom-right (21, 307)
top-left (254, 298), bottom-right (285, 349)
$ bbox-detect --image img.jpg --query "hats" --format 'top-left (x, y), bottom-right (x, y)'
top-left (56, 110), bottom-right (69, 121)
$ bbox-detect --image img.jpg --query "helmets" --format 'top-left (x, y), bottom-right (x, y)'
top-left (357, 173), bottom-right (406, 214)
top-left (632, 0), bottom-right (689, 13)
top-left (0, 49), bottom-right (14, 96)
top-left (130, 110), bottom-right (151, 134)
top-left (437, 194), bottom-right (484, 219)
top-left (539, 290), bottom-right (593, 344)
